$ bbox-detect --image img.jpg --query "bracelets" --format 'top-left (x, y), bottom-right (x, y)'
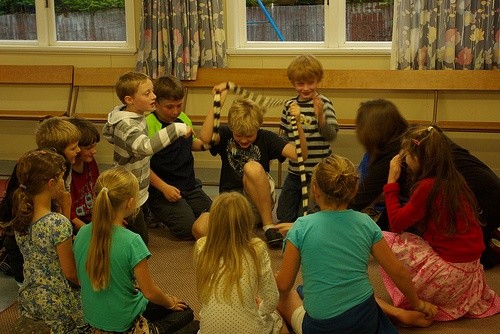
top-left (165, 295), bottom-right (177, 309)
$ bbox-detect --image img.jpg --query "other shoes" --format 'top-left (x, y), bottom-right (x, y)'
top-left (265, 228), bottom-right (284, 248)
top-left (173, 319), bottom-right (201, 334)
top-left (143, 204), bottom-right (158, 228)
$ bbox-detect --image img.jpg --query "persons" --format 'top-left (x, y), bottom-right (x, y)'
top-left (191, 192), bottom-right (290, 334)
top-left (199, 82), bottom-right (309, 247)
top-left (276, 54), bottom-right (339, 224)
top-left (0, 148), bottom-right (91, 334)
top-left (102, 71), bottom-right (196, 248)
top-left (74, 167), bottom-right (195, 334)
top-left (0, 112), bottom-right (102, 288)
top-left (139, 75), bottom-right (215, 241)
top-left (376, 123), bottom-right (500, 322)
top-left (275, 154), bottom-right (439, 334)
top-left (274, 100), bottom-right (500, 271)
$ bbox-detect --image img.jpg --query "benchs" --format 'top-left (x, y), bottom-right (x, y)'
top-left (0, 64), bottom-right (500, 187)
